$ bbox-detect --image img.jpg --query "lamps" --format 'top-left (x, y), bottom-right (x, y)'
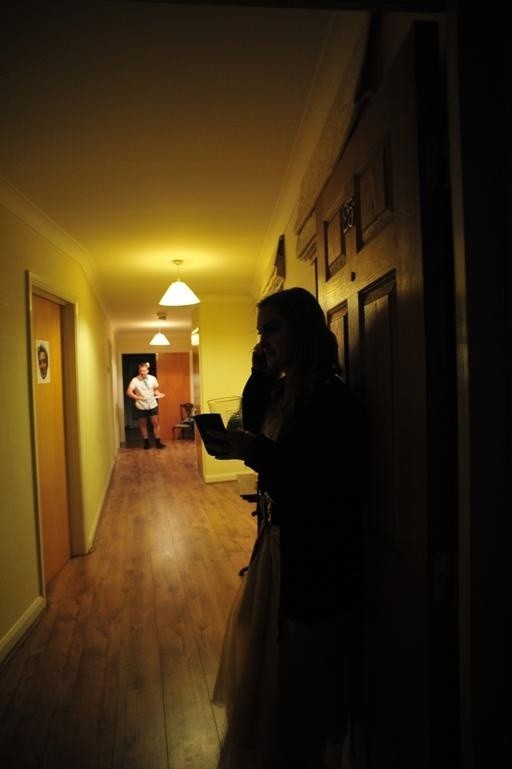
top-left (149, 314), bottom-right (171, 346)
top-left (158, 260), bottom-right (200, 307)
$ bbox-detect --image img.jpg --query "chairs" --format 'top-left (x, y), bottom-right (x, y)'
top-left (172, 402), bottom-right (194, 440)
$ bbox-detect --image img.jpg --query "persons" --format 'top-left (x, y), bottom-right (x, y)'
top-left (127, 362), bottom-right (165, 449)
top-left (193, 287), bottom-right (369, 769)
top-left (38, 346), bottom-right (49, 379)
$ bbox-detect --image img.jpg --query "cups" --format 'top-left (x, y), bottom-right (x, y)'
top-left (207, 396), bottom-right (244, 431)
